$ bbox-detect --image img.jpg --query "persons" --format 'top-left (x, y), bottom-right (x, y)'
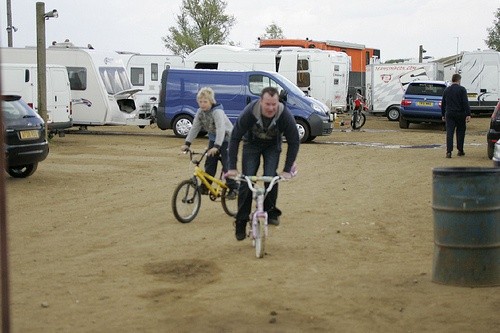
top-left (228, 87), bottom-right (300, 241)
top-left (181, 87), bottom-right (233, 195)
top-left (441, 74), bottom-right (470, 158)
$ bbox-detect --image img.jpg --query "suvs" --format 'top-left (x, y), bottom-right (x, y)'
top-left (399, 80), bottom-right (452, 131)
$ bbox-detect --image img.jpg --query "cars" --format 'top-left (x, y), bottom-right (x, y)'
top-left (0, 93), bottom-right (50, 179)
top-left (487, 98), bottom-right (500, 161)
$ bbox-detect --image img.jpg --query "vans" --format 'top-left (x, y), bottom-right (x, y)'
top-left (156, 68), bottom-right (334, 145)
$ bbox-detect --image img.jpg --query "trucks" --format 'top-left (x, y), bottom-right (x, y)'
top-left (365, 47), bottom-right (500, 122)
top-left (0, 43), bottom-right (352, 140)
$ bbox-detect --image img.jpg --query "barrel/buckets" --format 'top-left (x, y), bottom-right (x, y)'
top-left (431, 166), bottom-right (500, 287)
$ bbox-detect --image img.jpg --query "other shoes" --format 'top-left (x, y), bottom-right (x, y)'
top-left (228, 190), bottom-right (236, 199)
top-left (457, 151), bottom-right (465, 156)
top-left (446, 152), bottom-right (451, 158)
top-left (195, 186), bottom-right (207, 195)
top-left (268, 217), bottom-right (280, 226)
top-left (234, 220), bottom-right (246, 240)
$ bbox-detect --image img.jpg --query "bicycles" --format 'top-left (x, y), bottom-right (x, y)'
top-left (171, 147), bottom-right (239, 223)
top-left (222, 169), bottom-right (298, 259)
top-left (350, 92), bottom-right (370, 129)
